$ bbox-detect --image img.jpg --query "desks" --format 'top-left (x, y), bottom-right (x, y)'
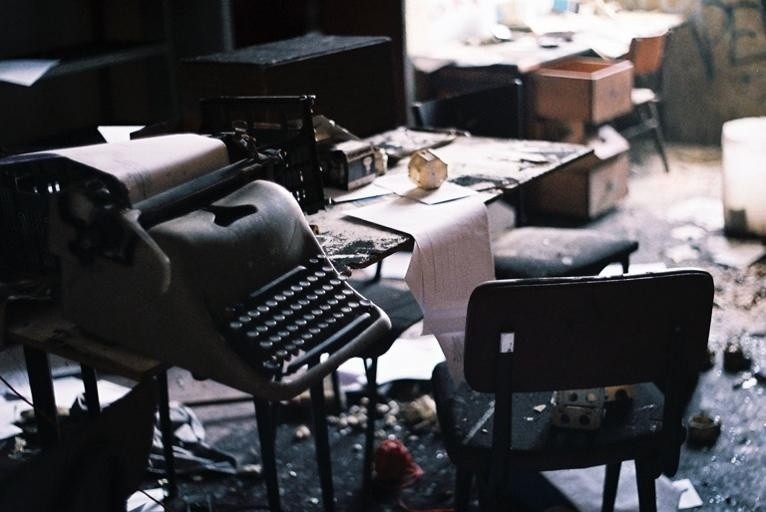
top-left (413, 8), bottom-right (689, 142)
top-left (0, 126), bottom-right (595, 512)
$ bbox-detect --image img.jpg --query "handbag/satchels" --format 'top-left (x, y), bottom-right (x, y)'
top-left (69, 394), bottom-right (205, 465)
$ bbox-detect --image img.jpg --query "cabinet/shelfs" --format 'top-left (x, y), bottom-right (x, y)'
top-left (178, 34), bottom-right (392, 152)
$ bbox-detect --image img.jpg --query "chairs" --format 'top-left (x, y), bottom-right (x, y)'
top-left (618, 31), bottom-right (672, 173)
top-left (408, 79), bottom-right (642, 274)
top-left (427, 268), bottom-right (716, 511)
